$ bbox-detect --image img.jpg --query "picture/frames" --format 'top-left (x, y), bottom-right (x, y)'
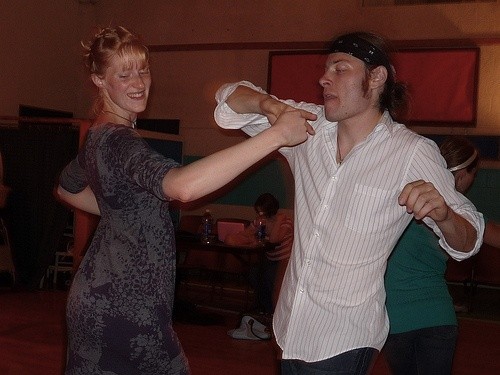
top-left (266, 48), bottom-right (480, 128)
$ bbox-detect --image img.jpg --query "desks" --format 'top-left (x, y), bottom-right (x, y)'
top-left (187, 241), bottom-right (278, 314)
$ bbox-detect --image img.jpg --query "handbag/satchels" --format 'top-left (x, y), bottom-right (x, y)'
top-left (227, 316), bottom-right (272, 342)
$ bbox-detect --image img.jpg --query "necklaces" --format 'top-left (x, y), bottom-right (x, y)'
top-left (336, 132), bottom-right (344, 166)
top-left (100, 110), bottom-right (137, 128)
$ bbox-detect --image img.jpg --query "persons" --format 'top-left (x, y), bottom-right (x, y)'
top-left (381, 135), bottom-right (500, 375)
top-left (56, 23), bottom-right (311, 375)
top-left (224, 192), bottom-right (294, 308)
top-left (213, 30), bottom-right (486, 375)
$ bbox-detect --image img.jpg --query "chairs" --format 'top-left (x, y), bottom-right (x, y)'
top-left (0, 219), bottom-right (16, 281)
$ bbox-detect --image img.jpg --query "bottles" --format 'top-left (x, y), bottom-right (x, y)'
top-left (256, 212), bottom-right (266, 244)
top-left (201, 209), bottom-right (216, 245)
